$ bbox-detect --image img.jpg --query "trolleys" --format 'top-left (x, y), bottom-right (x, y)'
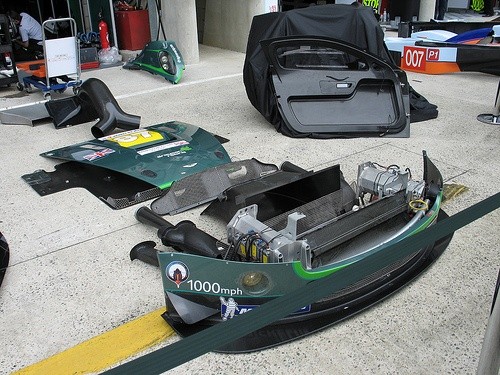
top-left (23, 17), bottom-right (82, 99)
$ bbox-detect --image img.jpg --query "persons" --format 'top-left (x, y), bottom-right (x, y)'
top-left (437, 0), bottom-right (449, 20)
top-left (479, 0), bottom-right (495, 17)
top-left (8, 11), bottom-right (46, 62)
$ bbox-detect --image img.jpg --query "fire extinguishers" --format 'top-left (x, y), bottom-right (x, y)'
top-left (99, 16), bottom-right (111, 49)
